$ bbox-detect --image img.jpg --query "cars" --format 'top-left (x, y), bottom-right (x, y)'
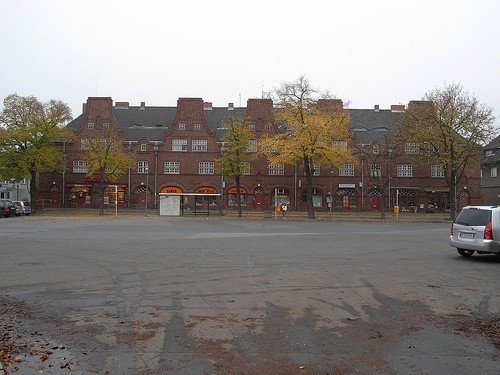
top-left (0, 198), bottom-right (16, 218)
top-left (450, 204), bottom-right (500, 263)
top-left (13, 200), bottom-right (32, 217)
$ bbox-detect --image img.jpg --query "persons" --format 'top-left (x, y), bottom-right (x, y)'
top-left (277, 203), bottom-right (288, 220)
